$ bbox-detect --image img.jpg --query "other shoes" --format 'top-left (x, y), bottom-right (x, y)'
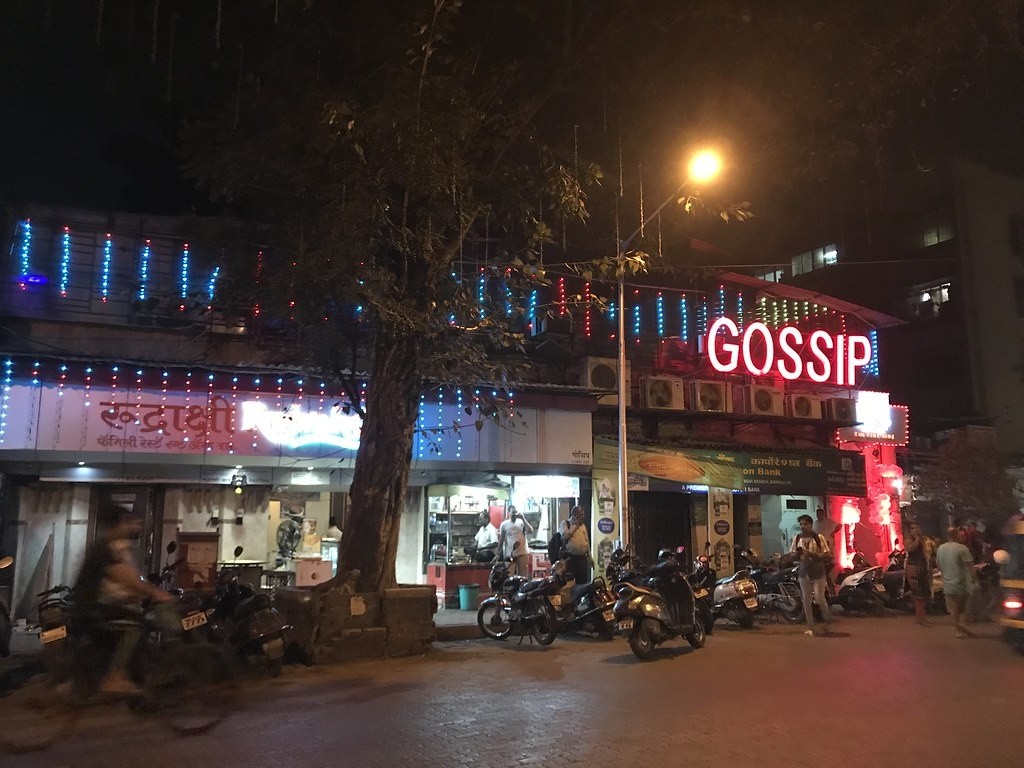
top-left (99, 676), bottom-right (143, 693)
top-left (916, 616), bottom-right (936, 627)
top-left (955, 631), bottom-right (962, 637)
top-left (803, 627), bottom-right (817, 637)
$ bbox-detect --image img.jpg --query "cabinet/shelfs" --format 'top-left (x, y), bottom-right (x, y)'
top-left (428, 513), bottom-right (480, 563)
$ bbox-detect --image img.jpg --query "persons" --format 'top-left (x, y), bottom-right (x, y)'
top-left (494, 505), bottom-right (533, 577)
top-left (958, 521), bottom-right (982, 548)
top-left (791, 515), bottom-right (833, 638)
top-left (329, 517), bottom-right (342, 539)
top-left (559, 506), bottom-right (594, 584)
top-left (903, 521), bottom-right (931, 626)
top-left (470, 512), bottom-right (498, 554)
top-left (812, 509), bottom-right (843, 552)
top-left (937, 526), bottom-right (975, 638)
top-left (62, 505), bottom-right (174, 701)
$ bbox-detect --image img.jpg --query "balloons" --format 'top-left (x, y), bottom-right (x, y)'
top-left (850, 464), bottom-right (902, 532)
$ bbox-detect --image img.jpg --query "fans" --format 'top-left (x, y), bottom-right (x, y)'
top-left (276, 518), bottom-right (301, 559)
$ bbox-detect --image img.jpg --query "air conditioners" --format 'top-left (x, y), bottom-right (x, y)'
top-left (827, 398), bottom-right (857, 422)
top-left (575, 355), bottom-right (633, 407)
top-left (743, 384), bottom-right (784, 417)
top-left (637, 375), bottom-right (685, 410)
top-left (689, 379), bottom-right (733, 414)
top-left (785, 393), bottom-right (823, 420)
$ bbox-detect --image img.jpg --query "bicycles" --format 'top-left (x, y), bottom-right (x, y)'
top-left (0, 587), bottom-right (240, 755)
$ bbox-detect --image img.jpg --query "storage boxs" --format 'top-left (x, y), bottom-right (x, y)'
top-left (179, 541), bottom-right (218, 564)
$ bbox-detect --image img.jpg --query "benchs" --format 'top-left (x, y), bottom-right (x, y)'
top-left (262, 570), bottom-right (295, 585)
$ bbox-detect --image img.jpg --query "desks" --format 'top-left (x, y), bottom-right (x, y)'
top-left (217, 559), bottom-right (269, 583)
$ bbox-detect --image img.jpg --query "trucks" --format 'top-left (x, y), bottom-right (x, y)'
top-left (995, 517), bottom-right (1024, 638)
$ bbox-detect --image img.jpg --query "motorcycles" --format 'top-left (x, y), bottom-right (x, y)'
top-left (687, 540), bottom-right (761, 630)
top-left (145, 539), bottom-right (296, 681)
top-left (606, 543), bottom-right (652, 598)
top-left (737, 545), bottom-right (834, 623)
top-left (477, 542), bottom-right (568, 644)
top-left (824, 538), bottom-right (998, 615)
top-left (542, 555), bottom-right (617, 642)
top-left (0, 555), bottom-right (16, 660)
top-left (25, 584), bottom-right (101, 681)
top-left (610, 545), bottom-right (714, 660)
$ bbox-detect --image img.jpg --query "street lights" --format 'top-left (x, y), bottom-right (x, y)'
top-left (614, 149), bottom-right (724, 545)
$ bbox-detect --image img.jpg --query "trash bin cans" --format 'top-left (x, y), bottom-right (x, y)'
top-left (458, 582), bottom-right (480, 611)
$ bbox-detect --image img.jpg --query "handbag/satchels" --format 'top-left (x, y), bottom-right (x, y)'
top-left (547, 519), bottom-right (571, 563)
